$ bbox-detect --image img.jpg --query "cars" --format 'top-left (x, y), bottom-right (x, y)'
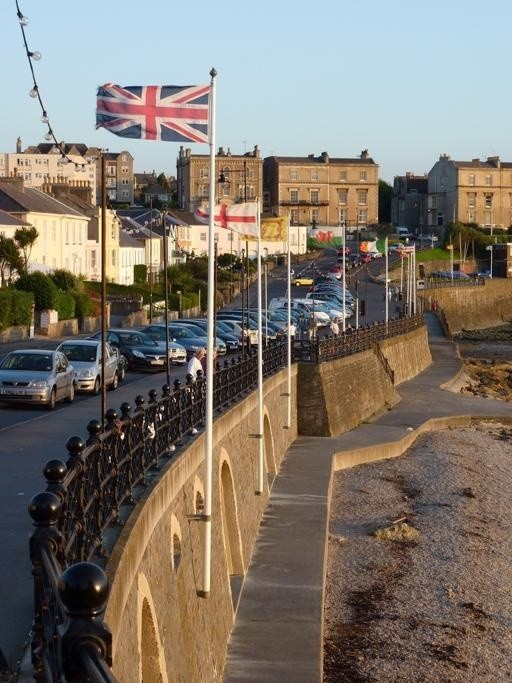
top-left (474, 271), bottom-right (491, 278)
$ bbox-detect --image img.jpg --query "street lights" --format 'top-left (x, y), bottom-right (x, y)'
top-left (217, 160), bottom-right (249, 350)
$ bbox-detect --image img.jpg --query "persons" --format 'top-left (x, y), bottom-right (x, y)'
top-left (201, 347), bottom-right (217, 374)
top-left (296, 310), bottom-right (307, 339)
top-left (186, 346), bottom-right (205, 434)
top-left (329, 316), bottom-right (341, 337)
top-left (284, 319), bottom-right (297, 341)
top-left (307, 312), bottom-right (318, 340)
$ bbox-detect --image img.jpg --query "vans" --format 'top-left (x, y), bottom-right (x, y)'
top-left (397, 226), bottom-right (413, 240)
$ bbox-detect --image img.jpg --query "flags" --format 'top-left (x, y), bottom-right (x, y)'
top-left (309, 227), bottom-right (343, 248)
top-left (193, 202), bottom-right (259, 235)
top-left (358, 238), bottom-right (385, 252)
top-left (239, 216), bottom-right (288, 242)
top-left (485, 245), bottom-right (492, 250)
top-left (94, 83), bottom-right (210, 143)
top-left (394, 242), bottom-right (414, 257)
top-left (446, 243), bottom-right (453, 249)
